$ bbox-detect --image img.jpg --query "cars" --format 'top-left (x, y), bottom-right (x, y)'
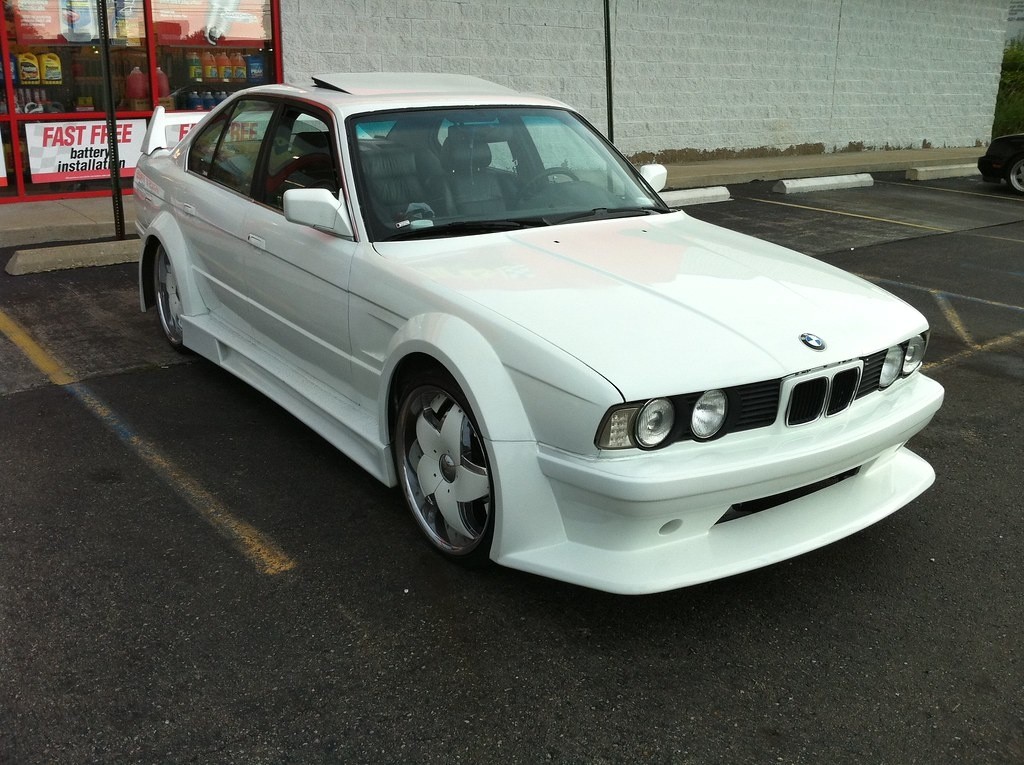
top-left (131, 70), bottom-right (945, 596)
top-left (977, 133), bottom-right (1024, 194)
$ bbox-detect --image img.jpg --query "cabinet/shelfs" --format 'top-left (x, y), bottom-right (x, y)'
top-left (0, 0), bottom-right (278, 113)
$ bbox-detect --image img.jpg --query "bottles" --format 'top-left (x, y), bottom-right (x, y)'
top-left (184, 52), bottom-right (266, 111)
top-left (156, 67), bottom-right (170, 98)
top-left (0, 51), bottom-right (63, 114)
top-left (125, 66), bottom-right (150, 98)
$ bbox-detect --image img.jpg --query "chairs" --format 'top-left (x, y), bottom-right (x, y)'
top-left (442, 136), bottom-right (523, 216)
top-left (366, 150), bottom-right (425, 223)
top-left (292, 132), bottom-right (335, 177)
